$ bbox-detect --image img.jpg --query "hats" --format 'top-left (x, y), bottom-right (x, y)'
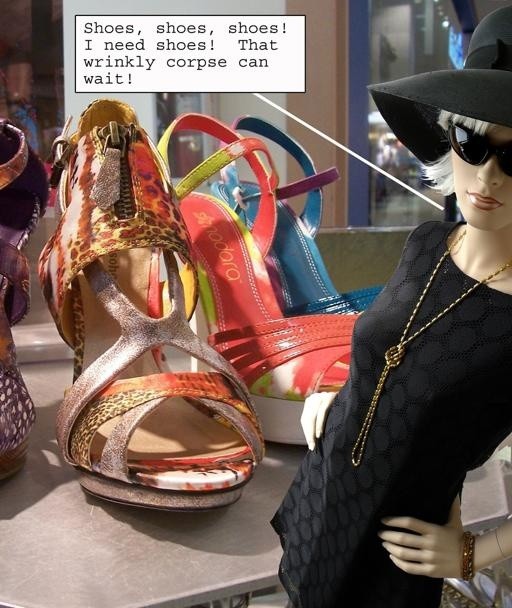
top-left (364, 3), bottom-right (512, 165)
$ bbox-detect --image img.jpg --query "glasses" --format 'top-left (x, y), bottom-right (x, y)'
top-left (445, 121), bottom-right (511, 177)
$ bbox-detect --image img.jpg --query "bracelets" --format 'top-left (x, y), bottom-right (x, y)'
top-left (493, 526), bottom-right (509, 560)
top-left (458, 529), bottom-right (477, 586)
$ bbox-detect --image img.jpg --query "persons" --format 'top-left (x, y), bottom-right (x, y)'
top-left (267, 4), bottom-right (512, 608)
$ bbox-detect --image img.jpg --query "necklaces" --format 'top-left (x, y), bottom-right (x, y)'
top-left (352, 224), bottom-right (510, 466)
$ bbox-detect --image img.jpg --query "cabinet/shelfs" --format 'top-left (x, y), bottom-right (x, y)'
top-left (1, 320), bottom-right (512, 608)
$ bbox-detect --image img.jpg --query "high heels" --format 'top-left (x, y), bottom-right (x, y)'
top-left (0, 117), bottom-right (375, 513)
